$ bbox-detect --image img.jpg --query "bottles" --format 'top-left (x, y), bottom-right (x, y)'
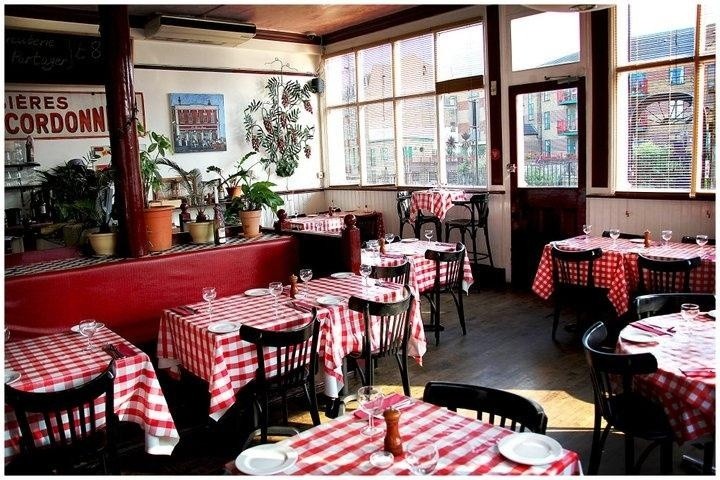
top-left (217, 178), bottom-right (225, 201)
top-left (213, 203), bottom-right (226, 244)
top-left (382, 404), bottom-right (402, 456)
top-left (30, 188), bottom-right (53, 224)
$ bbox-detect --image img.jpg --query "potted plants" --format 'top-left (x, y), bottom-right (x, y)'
top-left (206, 150), bottom-right (257, 200)
top-left (186, 166), bottom-right (217, 244)
top-left (222, 181), bottom-right (285, 237)
top-left (19, 147), bottom-right (117, 258)
top-left (135, 118), bottom-right (191, 251)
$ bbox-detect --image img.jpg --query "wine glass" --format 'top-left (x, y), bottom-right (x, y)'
top-left (357, 386), bottom-right (385, 438)
top-left (80, 320), bottom-right (98, 351)
top-left (428, 181), bottom-right (448, 192)
top-left (405, 441), bottom-right (438, 475)
top-left (583, 225), bottom-right (709, 255)
top-left (681, 304), bottom-right (699, 336)
top-left (292, 203), bottom-right (372, 221)
top-left (366, 229), bottom-right (434, 258)
top-left (202, 263), bottom-right (372, 332)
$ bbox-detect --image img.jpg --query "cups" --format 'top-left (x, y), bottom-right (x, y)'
top-left (4, 134), bottom-right (40, 187)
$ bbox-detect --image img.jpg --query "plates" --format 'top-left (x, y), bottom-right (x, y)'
top-left (234, 443), bottom-right (298, 476)
top-left (497, 432), bottom-right (565, 466)
top-left (620, 330), bottom-right (657, 344)
top-left (4, 368), bottom-right (21, 386)
top-left (70, 322), bottom-right (104, 334)
